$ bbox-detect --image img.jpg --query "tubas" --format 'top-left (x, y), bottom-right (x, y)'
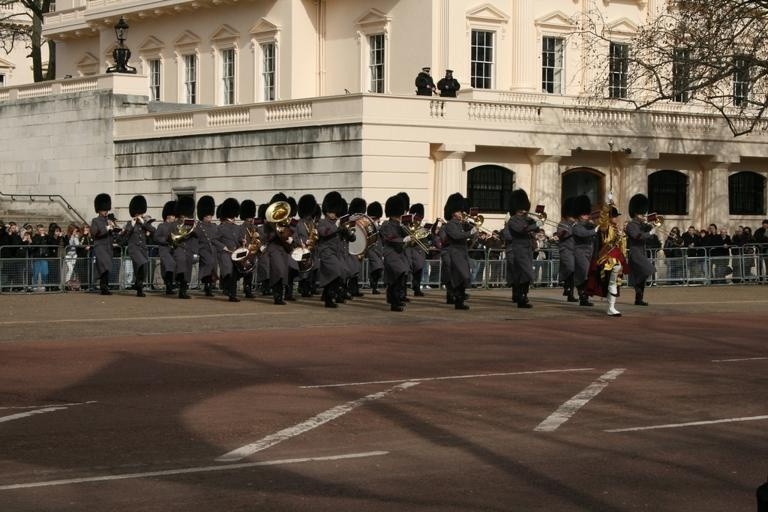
top-left (265, 201), bottom-right (293, 253)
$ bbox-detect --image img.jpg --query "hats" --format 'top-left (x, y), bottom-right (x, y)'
top-left (609, 207), bottom-right (618, 217)
top-left (444, 193), bottom-right (471, 220)
top-left (629, 193), bottom-right (648, 217)
top-left (385, 192), bottom-right (410, 218)
top-left (409, 202), bottom-right (424, 221)
top-left (562, 195), bottom-right (591, 218)
top-left (509, 192), bottom-right (530, 216)
top-left (367, 201), bottom-right (383, 219)
top-left (162, 197), bottom-right (196, 224)
top-left (129, 195), bottom-right (147, 217)
top-left (258, 191), bottom-right (348, 221)
top-left (197, 195), bottom-right (215, 221)
top-left (216, 198), bottom-right (240, 219)
top-left (348, 198), bottom-right (366, 216)
top-left (240, 200), bottom-right (256, 221)
top-left (94, 193), bottom-right (112, 213)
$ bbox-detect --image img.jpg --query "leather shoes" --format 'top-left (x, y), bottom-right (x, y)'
top-left (565, 288), bottom-right (593, 306)
top-left (512, 296), bottom-right (530, 308)
top-left (635, 300), bottom-right (646, 305)
top-left (387, 291), bottom-right (423, 311)
top-left (101, 283), bottom-right (380, 308)
top-left (447, 294), bottom-right (469, 309)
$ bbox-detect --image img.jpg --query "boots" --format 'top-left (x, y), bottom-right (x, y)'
top-left (607, 290), bottom-right (621, 315)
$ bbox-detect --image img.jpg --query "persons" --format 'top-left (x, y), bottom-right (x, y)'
top-left (415, 67), bottom-right (437, 96)
top-left (437, 69), bottom-right (461, 97)
top-left (0, 188), bottom-right (768, 318)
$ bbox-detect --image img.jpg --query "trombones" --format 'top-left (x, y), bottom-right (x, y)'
top-left (459, 213), bottom-right (500, 241)
top-left (639, 215), bottom-right (677, 239)
top-left (525, 212), bottom-right (569, 232)
top-left (395, 217), bottom-right (430, 254)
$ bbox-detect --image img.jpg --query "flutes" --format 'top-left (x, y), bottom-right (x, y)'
top-left (133, 216), bottom-right (144, 220)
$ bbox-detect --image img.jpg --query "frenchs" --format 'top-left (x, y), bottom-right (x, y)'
top-left (170, 218), bottom-right (196, 243)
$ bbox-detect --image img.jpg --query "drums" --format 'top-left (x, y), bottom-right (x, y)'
top-left (290, 247), bottom-right (313, 272)
top-left (230, 247), bottom-right (256, 274)
top-left (341, 212), bottom-right (378, 256)
